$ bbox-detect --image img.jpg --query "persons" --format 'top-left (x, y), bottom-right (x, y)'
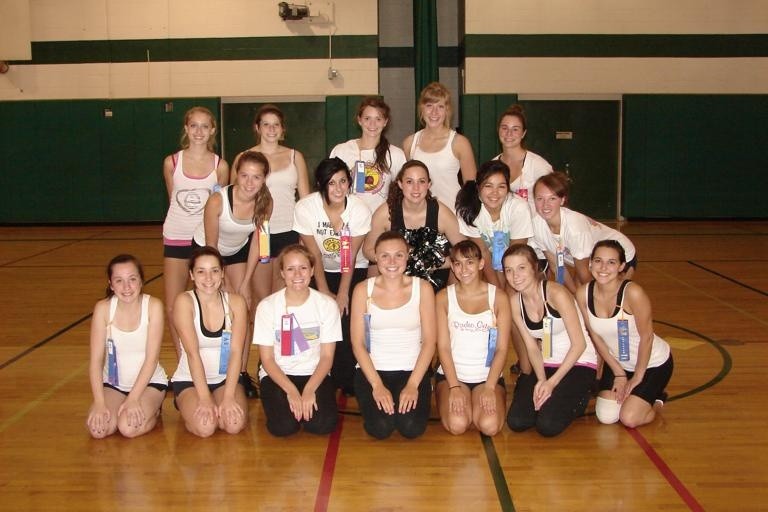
top-left (159, 151), bottom-right (596, 398)
top-left (252, 243), bottom-right (345, 438)
top-left (230, 107), bottom-right (310, 258)
top-left (329, 97), bottom-right (407, 216)
top-left (403, 81), bottom-right (477, 216)
top-left (574, 240), bottom-right (673, 428)
top-left (161, 107), bottom-right (229, 362)
top-left (502, 250), bottom-right (597, 442)
top-left (533, 172), bottom-right (638, 293)
top-left (169, 246), bottom-right (249, 438)
top-left (433, 240), bottom-right (512, 438)
top-left (348, 229), bottom-right (438, 441)
top-left (84, 253), bottom-right (170, 440)
top-left (492, 105), bottom-right (553, 218)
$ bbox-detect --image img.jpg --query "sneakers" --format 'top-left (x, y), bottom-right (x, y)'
top-left (343, 386), bottom-right (354, 397)
top-left (240, 372), bottom-right (257, 398)
